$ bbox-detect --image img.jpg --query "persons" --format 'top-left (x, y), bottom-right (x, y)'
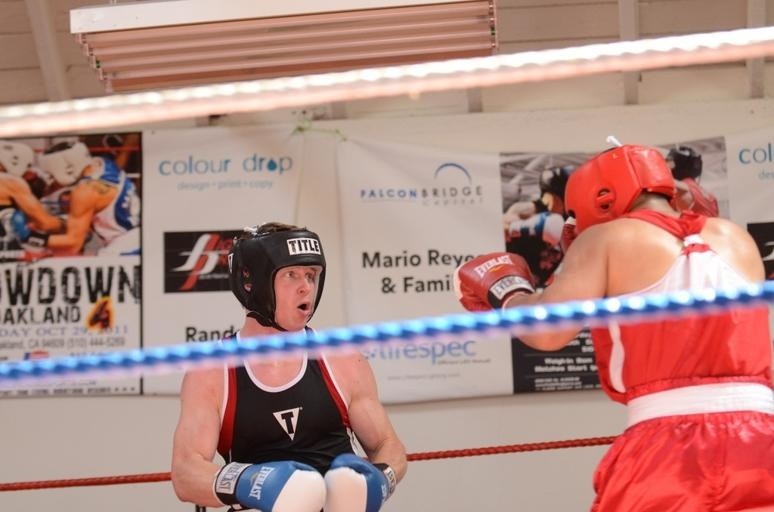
top-left (0, 131), bottom-right (143, 265)
top-left (168, 219), bottom-right (408, 512)
top-left (451, 141), bottom-right (774, 512)
top-left (662, 143), bottom-right (722, 221)
top-left (501, 163), bottom-right (578, 286)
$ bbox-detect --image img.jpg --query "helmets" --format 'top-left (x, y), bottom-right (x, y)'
top-left (228, 222), bottom-right (326, 333)
top-left (665, 146), bottom-right (702, 180)
top-left (539, 167), bottom-right (568, 197)
top-left (564, 144), bottom-right (675, 232)
top-left (0, 138), bottom-right (95, 187)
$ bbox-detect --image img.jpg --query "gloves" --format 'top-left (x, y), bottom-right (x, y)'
top-left (1, 208), bottom-right (49, 251)
top-left (454, 251), bottom-right (536, 318)
top-left (509, 211), bottom-right (564, 246)
top-left (563, 216), bottom-right (577, 245)
top-left (212, 453), bottom-right (397, 512)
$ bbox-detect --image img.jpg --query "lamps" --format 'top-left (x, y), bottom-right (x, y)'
top-left (70, 0), bottom-right (499, 94)
